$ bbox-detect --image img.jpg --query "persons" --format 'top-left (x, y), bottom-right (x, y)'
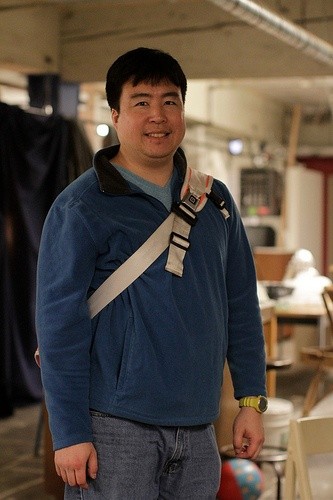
top-left (32, 47), bottom-right (266, 500)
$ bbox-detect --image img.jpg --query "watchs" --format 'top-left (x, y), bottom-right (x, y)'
top-left (237, 396), bottom-right (267, 413)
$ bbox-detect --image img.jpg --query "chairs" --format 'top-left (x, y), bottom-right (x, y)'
top-left (299, 286), bottom-right (333, 416)
